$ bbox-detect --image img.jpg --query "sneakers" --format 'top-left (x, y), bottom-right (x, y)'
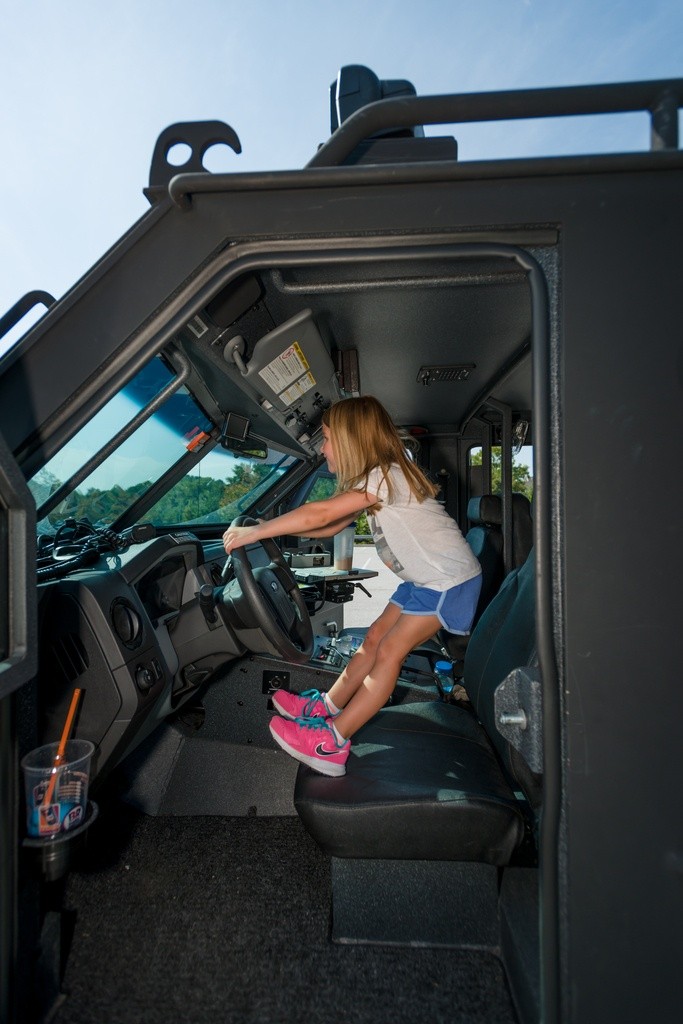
top-left (271, 686), bottom-right (332, 722)
top-left (269, 714), bottom-right (352, 777)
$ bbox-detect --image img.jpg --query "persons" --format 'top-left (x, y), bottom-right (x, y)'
top-left (223, 394), bottom-right (483, 776)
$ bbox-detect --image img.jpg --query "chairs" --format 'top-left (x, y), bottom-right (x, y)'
top-left (292, 494), bottom-right (543, 866)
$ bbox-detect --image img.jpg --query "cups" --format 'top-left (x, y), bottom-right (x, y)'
top-left (21, 739), bottom-right (96, 838)
top-left (334, 522), bottom-right (356, 570)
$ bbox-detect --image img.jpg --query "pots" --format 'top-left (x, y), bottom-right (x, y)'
top-left (18, 795), bottom-right (99, 882)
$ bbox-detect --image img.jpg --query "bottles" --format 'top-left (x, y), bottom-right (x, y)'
top-left (434, 661), bottom-right (455, 694)
top-left (330, 636), bottom-right (364, 655)
